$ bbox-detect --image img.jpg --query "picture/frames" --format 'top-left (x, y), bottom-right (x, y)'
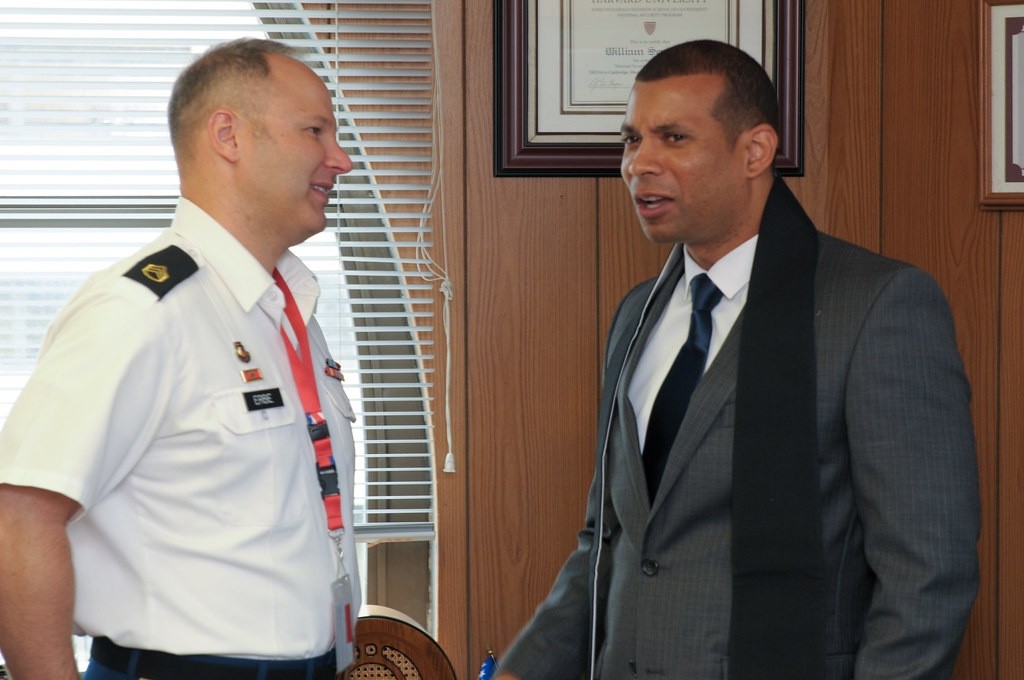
top-left (492, 0), bottom-right (808, 178)
top-left (972, 0), bottom-right (1024, 212)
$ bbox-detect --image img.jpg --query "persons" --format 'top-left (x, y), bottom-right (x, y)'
top-left (487, 39), bottom-right (980, 680)
top-left (0, 38), bottom-right (361, 680)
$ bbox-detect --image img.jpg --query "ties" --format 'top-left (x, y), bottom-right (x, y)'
top-left (643, 273), bottom-right (724, 503)
